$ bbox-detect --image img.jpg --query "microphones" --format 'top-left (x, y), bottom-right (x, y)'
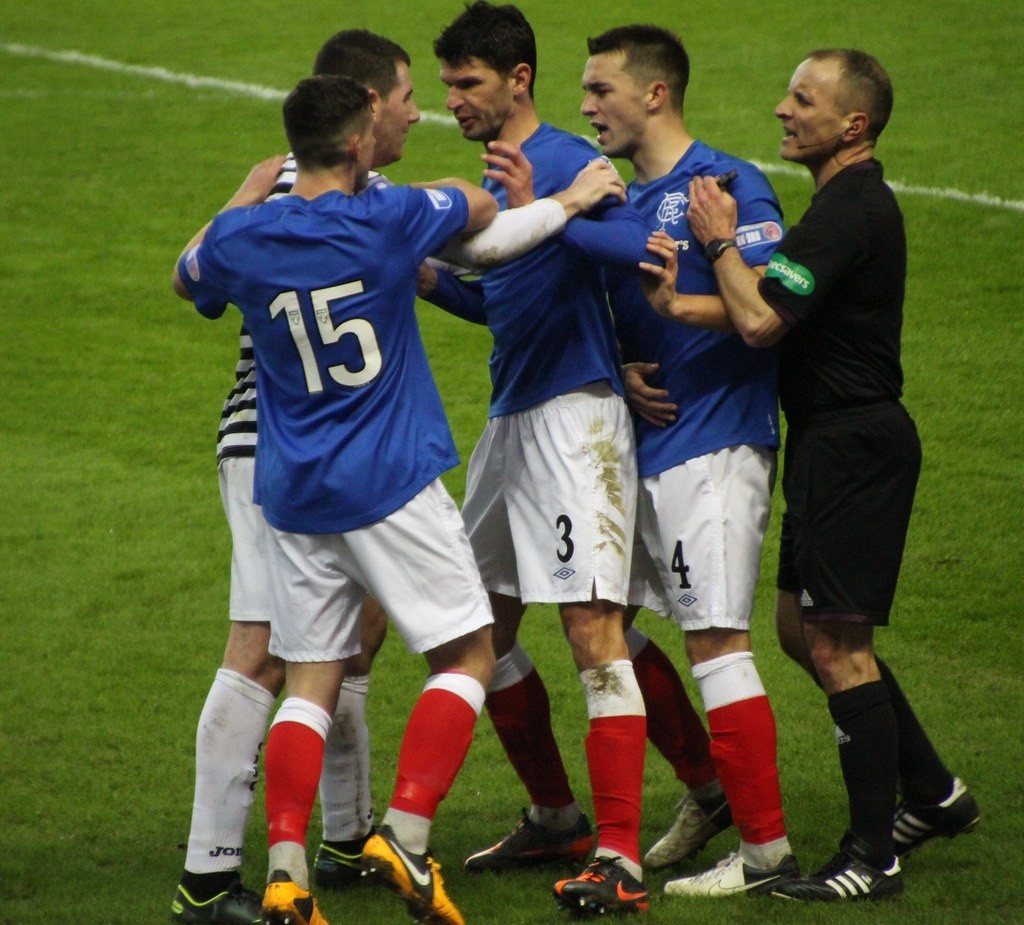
top-left (796, 127), bottom-right (853, 149)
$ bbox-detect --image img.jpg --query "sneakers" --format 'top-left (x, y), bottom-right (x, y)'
top-left (170, 885), bottom-right (264, 925)
top-left (259, 870), bottom-right (329, 925)
top-left (312, 844), bottom-right (374, 886)
top-left (644, 794), bottom-right (734, 869)
top-left (769, 851), bottom-right (903, 903)
top-left (553, 854), bottom-right (649, 914)
top-left (891, 776), bottom-right (980, 856)
top-left (464, 807), bottom-right (594, 873)
top-left (360, 823), bottom-right (466, 925)
top-left (662, 853), bottom-right (799, 895)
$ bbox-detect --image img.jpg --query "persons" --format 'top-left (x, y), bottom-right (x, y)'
top-left (169, 0), bottom-right (798, 925)
top-left (686, 46), bottom-right (981, 902)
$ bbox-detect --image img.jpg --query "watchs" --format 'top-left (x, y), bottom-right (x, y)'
top-left (705, 237), bottom-right (737, 263)
